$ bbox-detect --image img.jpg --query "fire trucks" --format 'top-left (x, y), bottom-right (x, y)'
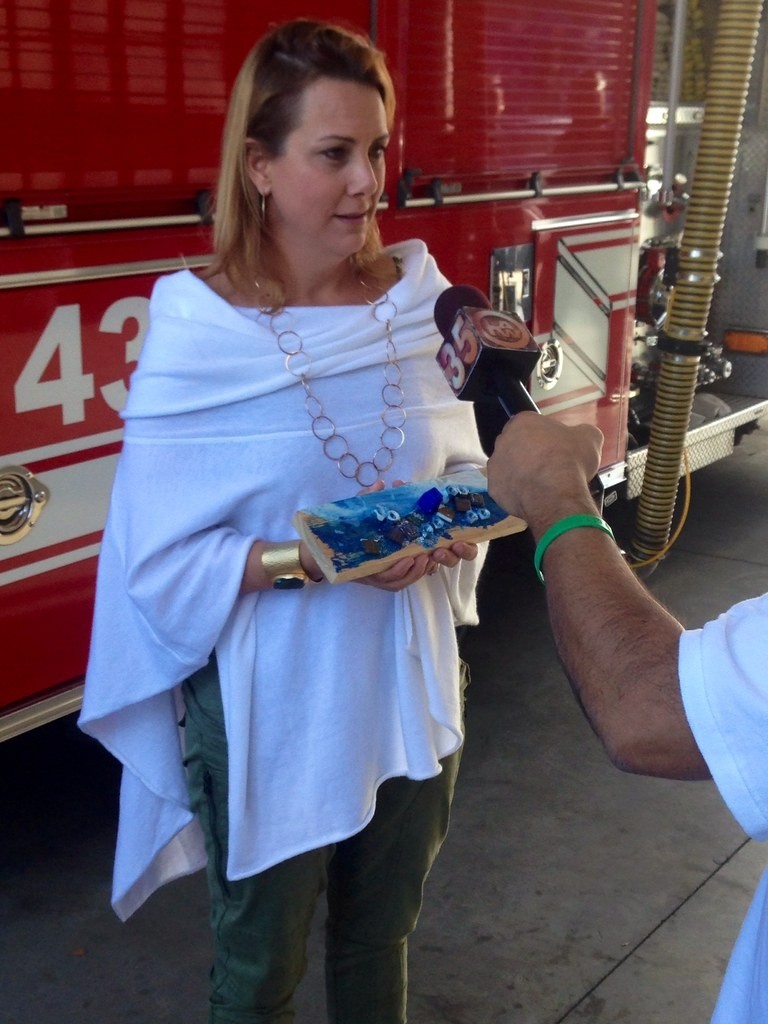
top-left (1, 1), bottom-right (768, 743)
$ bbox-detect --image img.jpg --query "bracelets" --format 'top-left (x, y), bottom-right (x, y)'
top-left (534, 515), bottom-right (615, 585)
top-left (261, 540), bottom-right (323, 589)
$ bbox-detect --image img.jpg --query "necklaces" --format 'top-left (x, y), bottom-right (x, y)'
top-left (253, 260), bottom-right (408, 489)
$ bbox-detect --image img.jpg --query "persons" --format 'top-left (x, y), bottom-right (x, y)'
top-left (78, 22), bottom-right (492, 1024)
top-left (487, 411), bottom-right (768, 1024)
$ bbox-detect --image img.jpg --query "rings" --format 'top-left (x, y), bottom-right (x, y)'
top-left (428, 563), bottom-right (440, 576)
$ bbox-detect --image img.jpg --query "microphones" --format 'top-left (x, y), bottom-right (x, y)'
top-left (432, 283), bottom-right (543, 415)
